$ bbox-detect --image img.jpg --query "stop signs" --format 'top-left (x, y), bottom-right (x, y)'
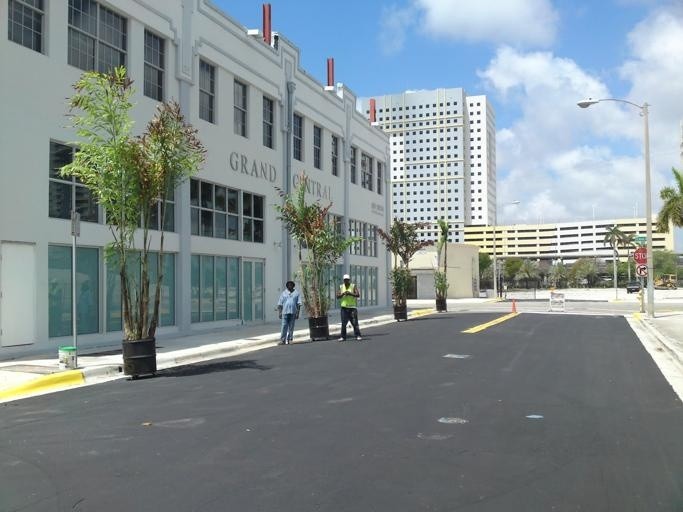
top-left (632, 248), bottom-right (647, 265)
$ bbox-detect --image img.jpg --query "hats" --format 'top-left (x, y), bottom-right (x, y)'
top-left (343, 273), bottom-right (350, 280)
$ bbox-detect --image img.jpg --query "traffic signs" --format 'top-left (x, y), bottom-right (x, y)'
top-left (634, 236), bottom-right (646, 242)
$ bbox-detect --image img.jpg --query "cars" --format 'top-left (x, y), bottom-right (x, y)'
top-left (625, 281), bottom-right (640, 294)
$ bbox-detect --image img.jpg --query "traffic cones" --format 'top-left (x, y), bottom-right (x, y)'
top-left (510, 300), bottom-right (518, 314)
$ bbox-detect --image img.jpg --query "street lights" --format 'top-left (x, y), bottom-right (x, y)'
top-left (491, 200), bottom-right (520, 300)
top-left (575, 98), bottom-right (655, 320)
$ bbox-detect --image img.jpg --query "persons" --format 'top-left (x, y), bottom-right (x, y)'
top-left (277, 280), bottom-right (302, 344)
top-left (336, 274), bottom-right (363, 341)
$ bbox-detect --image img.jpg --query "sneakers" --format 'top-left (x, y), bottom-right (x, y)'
top-left (337, 335), bottom-right (363, 341)
top-left (277, 338), bottom-right (294, 346)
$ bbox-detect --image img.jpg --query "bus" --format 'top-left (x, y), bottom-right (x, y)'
top-left (660, 274), bottom-right (676, 287)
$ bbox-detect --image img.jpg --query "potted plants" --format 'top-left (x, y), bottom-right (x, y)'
top-left (268, 172), bottom-right (367, 341)
top-left (53, 63), bottom-right (209, 379)
top-left (374, 217), bottom-right (449, 321)
